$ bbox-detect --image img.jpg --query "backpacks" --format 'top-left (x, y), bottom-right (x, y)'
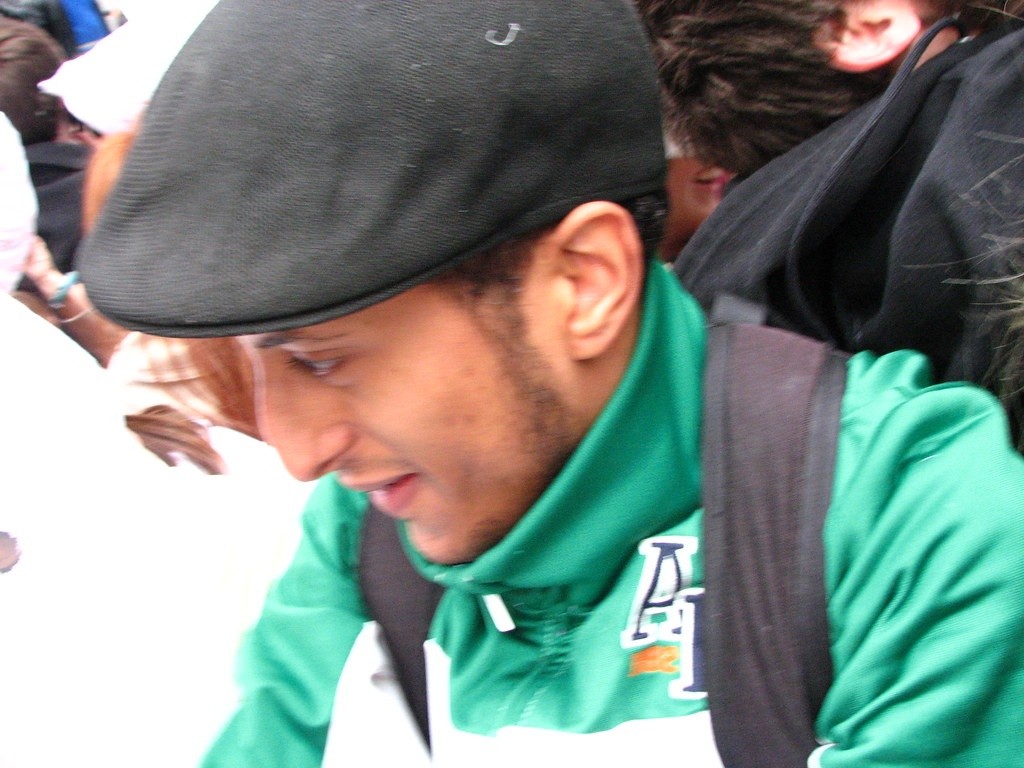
top-left (359, 12), bottom-right (1024, 768)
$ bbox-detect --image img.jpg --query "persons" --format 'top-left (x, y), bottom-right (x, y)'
top-left (2, 0), bottom-right (316, 767)
top-left (638, 0), bottom-right (1024, 461)
top-left (74, 1), bottom-right (1024, 767)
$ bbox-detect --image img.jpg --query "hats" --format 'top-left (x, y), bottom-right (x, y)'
top-left (81, 1), bottom-right (666, 338)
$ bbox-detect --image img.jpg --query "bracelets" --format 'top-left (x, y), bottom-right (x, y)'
top-left (49, 269), bottom-right (78, 311)
